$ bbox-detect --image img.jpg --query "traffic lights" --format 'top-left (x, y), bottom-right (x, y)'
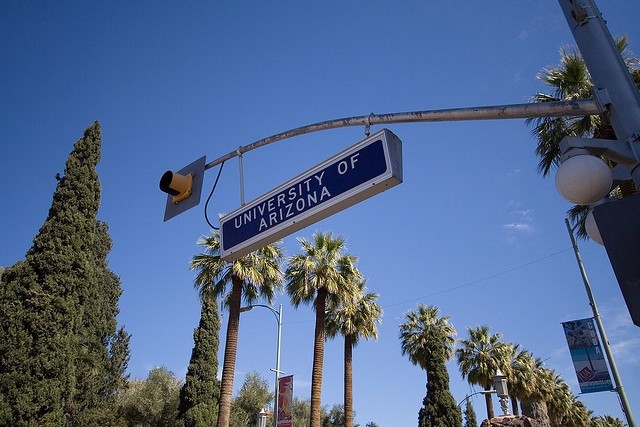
top-left (160, 98), bottom-right (601, 222)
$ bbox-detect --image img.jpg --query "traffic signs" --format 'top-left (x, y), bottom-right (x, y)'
top-left (217, 116), bottom-right (404, 260)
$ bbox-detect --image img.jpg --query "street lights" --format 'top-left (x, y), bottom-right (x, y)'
top-left (455, 390), bottom-right (497, 407)
top-left (239, 303), bottom-right (285, 426)
